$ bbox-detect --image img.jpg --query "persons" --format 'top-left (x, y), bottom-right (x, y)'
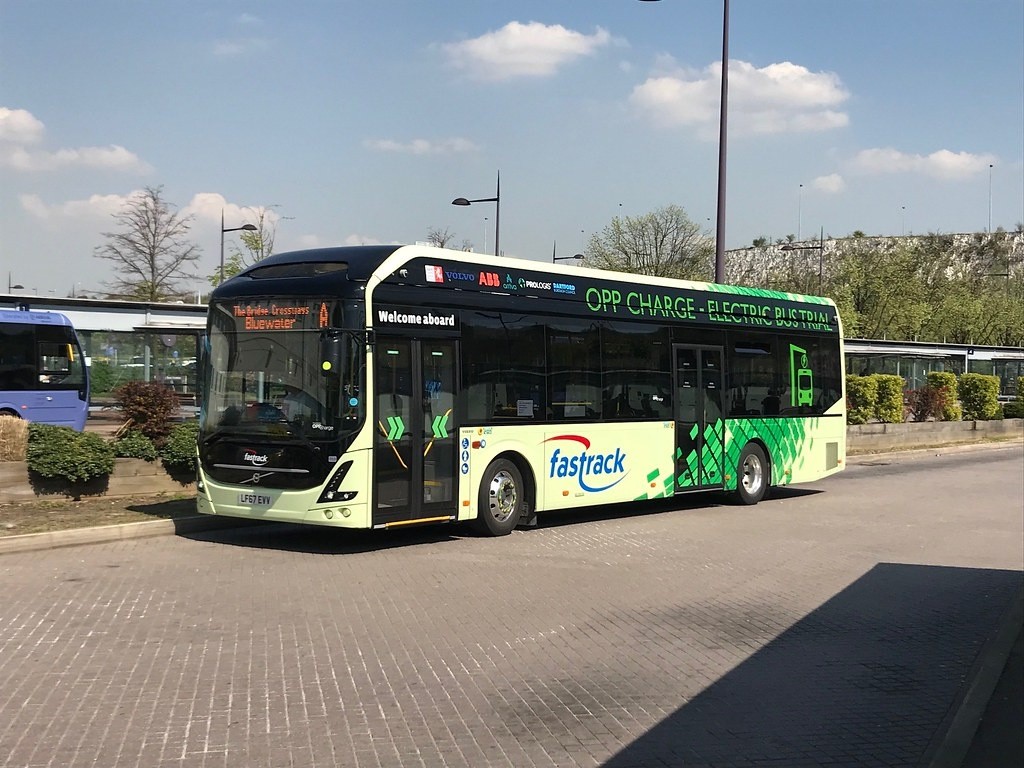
top-left (858, 368), bottom-right (870, 377)
top-left (281, 370), bottom-right (314, 421)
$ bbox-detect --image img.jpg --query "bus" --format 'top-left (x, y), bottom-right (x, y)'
top-left (0, 310), bottom-right (92, 435)
top-left (195, 245), bottom-right (847, 538)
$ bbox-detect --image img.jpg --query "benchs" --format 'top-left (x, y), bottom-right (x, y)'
top-left (167, 393), bottom-right (198, 423)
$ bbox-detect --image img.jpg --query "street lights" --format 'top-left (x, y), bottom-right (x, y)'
top-left (705, 218), bottom-right (713, 251)
top-left (551, 240), bottom-right (585, 265)
top-left (797, 185), bottom-right (803, 241)
top-left (618, 204), bottom-right (625, 265)
top-left (451, 168), bottom-right (500, 257)
top-left (989, 165), bottom-right (996, 233)
top-left (8, 271), bottom-right (26, 296)
top-left (902, 206), bottom-right (906, 236)
top-left (580, 230), bottom-right (586, 255)
top-left (483, 218), bottom-right (489, 256)
top-left (220, 209), bottom-right (257, 283)
